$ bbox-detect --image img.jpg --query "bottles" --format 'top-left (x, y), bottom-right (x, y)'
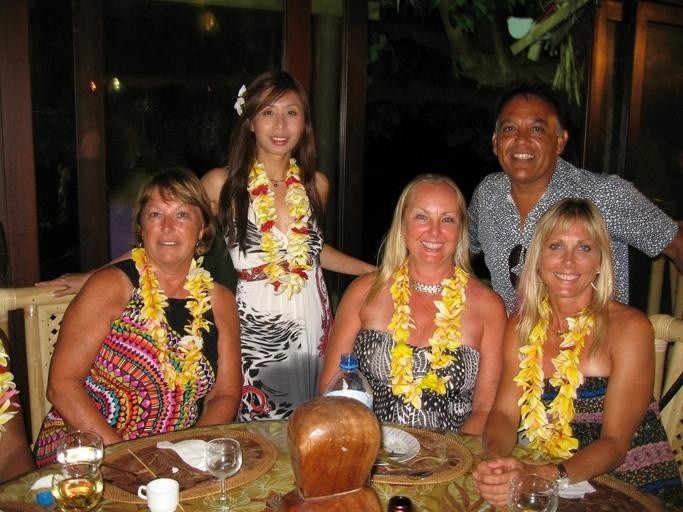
top-left (321, 354), bottom-right (374, 414)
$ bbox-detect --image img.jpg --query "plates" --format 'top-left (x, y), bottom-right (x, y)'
top-left (375, 426), bottom-right (421, 466)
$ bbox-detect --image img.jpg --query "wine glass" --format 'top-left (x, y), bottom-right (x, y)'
top-left (204, 438), bottom-right (244, 509)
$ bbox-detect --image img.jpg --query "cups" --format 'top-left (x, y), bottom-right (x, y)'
top-left (507, 475), bottom-right (558, 512)
top-left (139, 476), bottom-right (179, 512)
top-left (51, 432), bottom-right (104, 512)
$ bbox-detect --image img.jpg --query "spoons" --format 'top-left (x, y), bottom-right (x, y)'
top-left (99, 463), bottom-right (153, 484)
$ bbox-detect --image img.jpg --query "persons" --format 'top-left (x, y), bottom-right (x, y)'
top-left (319, 173), bottom-right (508, 435)
top-left (34, 66), bottom-right (380, 422)
top-left (466, 86), bottom-right (683, 318)
top-left (0, 337), bottom-right (38, 484)
top-left (32, 166), bottom-right (241, 471)
top-left (472, 198), bottom-right (683, 512)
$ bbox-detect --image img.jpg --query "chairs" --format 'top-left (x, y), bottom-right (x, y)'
top-left (2, 287), bottom-right (89, 447)
top-left (648, 311), bottom-right (682, 478)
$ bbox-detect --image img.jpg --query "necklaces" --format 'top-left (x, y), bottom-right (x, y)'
top-left (406, 271), bottom-right (454, 294)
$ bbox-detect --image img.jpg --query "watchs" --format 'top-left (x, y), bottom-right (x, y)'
top-left (556, 461), bottom-right (570, 491)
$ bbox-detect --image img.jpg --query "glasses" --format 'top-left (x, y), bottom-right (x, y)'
top-left (509, 244), bottom-right (522, 290)
